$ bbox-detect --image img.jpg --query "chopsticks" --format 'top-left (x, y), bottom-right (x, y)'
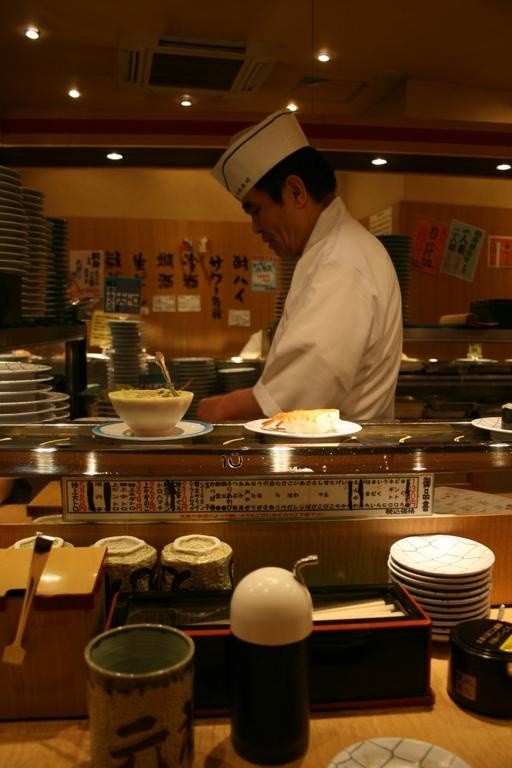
top-left (196, 599), bottom-right (403, 626)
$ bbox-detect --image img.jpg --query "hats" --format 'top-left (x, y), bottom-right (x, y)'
top-left (210, 108), bottom-right (310, 202)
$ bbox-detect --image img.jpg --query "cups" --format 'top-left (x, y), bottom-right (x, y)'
top-left (87, 534), bottom-right (158, 607)
top-left (82, 624), bottom-right (196, 767)
top-left (159, 533), bottom-right (234, 615)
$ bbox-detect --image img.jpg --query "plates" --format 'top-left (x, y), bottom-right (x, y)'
top-left (471, 417), bottom-right (512, 435)
top-left (243, 418), bottom-right (362, 439)
top-left (376, 233), bottom-right (411, 324)
top-left (172, 357), bottom-right (261, 415)
top-left (387, 535), bottom-right (496, 645)
top-left (0, 354), bottom-right (72, 425)
top-left (0, 165), bottom-right (69, 321)
top-left (326, 735), bottom-right (470, 767)
top-left (92, 419), bottom-right (213, 442)
top-left (269, 255), bottom-right (298, 321)
top-left (108, 319), bottom-right (147, 385)
top-left (93, 388), bottom-right (118, 419)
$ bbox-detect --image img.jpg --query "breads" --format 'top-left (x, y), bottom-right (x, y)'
top-left (261, 408), bottom-right (341, 434)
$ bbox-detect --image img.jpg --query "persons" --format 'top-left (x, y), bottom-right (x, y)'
top-left (190, 106), bottom-right (403, 425)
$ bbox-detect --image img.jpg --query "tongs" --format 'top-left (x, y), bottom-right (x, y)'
top-left (3, 536), bottom-right (58, 663)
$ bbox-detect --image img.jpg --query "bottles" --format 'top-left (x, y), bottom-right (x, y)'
top-left (229, 566), bottom-right (313, 764)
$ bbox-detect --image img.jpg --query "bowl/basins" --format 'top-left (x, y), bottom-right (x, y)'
top-left (108, 389), bottom-right (194, 435)
top-left (456, 356), bottom-right (497, 375)
top-left (469, 298), bottom-right (511, 328)
